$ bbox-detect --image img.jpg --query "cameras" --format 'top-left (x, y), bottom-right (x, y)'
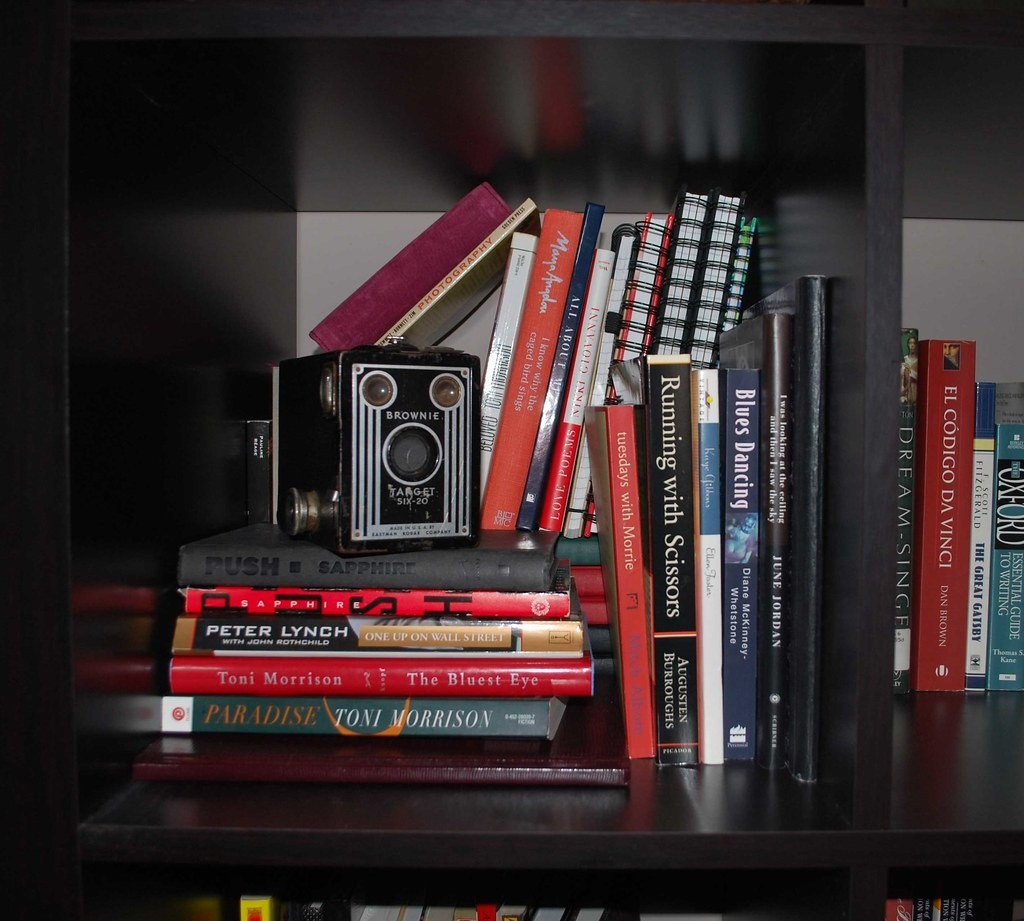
top-left (278, 337), bottom-right (481, 557)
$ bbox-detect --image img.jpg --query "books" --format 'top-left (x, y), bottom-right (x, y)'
top-left (133, 182), bottom-right (826, 785)
top-left (885, 896), bottom-right (1024, 921)
top-left (175, 883), bottom-right (723, 921)
top-left (893, 328), bottom-right (1023, 693)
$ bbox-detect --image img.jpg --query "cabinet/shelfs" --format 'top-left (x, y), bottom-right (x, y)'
top-left (78, 0), bottom-right (1023, 896)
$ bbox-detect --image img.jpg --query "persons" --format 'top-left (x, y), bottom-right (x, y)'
top-left (900, 346), bottom-right (910, 404)
top-left (904, 337), bottom-right (918, 404)
top-left (944, 344), bottom-right (959, 366)
